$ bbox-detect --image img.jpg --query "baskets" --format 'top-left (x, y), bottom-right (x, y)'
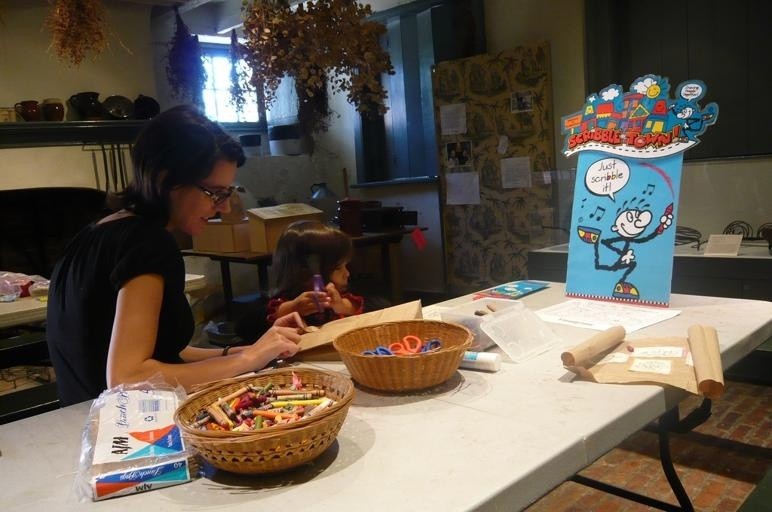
top-left (332, 318), bottom-right (476, 394)
top-left (172, 367), bottom-right (356, 476)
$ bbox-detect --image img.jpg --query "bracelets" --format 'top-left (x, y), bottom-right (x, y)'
top-left (222, 345), bottom-right (230, 355)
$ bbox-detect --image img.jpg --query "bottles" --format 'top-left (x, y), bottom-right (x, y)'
top-left (336, 197), bottom-right (361, 237)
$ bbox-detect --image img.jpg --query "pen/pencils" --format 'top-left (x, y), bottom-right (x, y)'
top-left (189, 382), bottom-right (338, 431)
top-left (487, 304), bottom-right (495, 312)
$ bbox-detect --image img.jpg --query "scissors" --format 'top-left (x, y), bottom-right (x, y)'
top-left (313, 274), bottom-right (328, 313)
top-left (361, 335), bottom-right (441, 355)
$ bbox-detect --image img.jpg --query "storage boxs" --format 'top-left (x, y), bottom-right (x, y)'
top-left (192, 218), bottom-right (249, 252)
top-left (247, 203), bottom-right (327, 253)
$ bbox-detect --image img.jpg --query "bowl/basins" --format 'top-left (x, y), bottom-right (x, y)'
top-left (133, 95), bottom-right (160, 120)
top-left (103, 95), bottom-right (133, 119)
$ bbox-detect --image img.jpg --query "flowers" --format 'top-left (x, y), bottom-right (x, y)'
top-left (40, 0), bottom-right (134, 72)
top-left (237, 0), bottom-right (396, 117)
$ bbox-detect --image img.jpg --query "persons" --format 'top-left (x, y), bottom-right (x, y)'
top-left (265, 219), bottom-right (364, 329)
top-left (46, 102), bottom-right (312, 408)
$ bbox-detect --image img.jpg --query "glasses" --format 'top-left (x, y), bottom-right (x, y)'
top-left (195, 181), bottom-right (233, 204)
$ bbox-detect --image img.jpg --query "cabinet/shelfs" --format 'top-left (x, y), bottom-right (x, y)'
top-left (528, 235), bottom-right (771, 386)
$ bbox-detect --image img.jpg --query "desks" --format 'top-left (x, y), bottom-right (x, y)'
top-left (1, 274), bottom-right (205, 424)
top-left (0, 279), bottom-right (771, 512)
top-left (179, 227), bottom-right (429, 320)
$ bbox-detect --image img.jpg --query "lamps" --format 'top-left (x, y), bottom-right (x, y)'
top-left (310, 182), bottom-right (338, 208)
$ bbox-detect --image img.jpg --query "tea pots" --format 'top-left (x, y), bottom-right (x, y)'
top-left (68, 92), bottom-right (103, 120)
top-left (14, 99), bottom-right (40, 120)
